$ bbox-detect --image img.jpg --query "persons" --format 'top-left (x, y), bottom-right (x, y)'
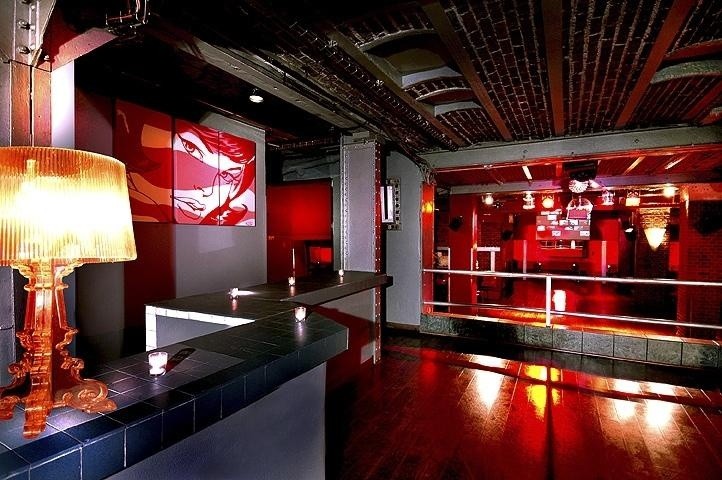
top-left (174, 118), bottom-right (256, 228)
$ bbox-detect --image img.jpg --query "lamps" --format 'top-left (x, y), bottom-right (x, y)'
top-left (484, 179), bottom-right (676, 208)
top-left (641, 210), bottom-right (670, 248)
top-left (0, 147), bottom-right (138, 440)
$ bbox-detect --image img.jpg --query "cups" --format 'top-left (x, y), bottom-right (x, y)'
top-left (228, 288), bottom-right (238, 299)
top-left (295, 306), bottom-right (306, 323)
top-left (288, 277), bottom-right (296, 286)
top-left (148, 352), bottom-right (168, 378)
